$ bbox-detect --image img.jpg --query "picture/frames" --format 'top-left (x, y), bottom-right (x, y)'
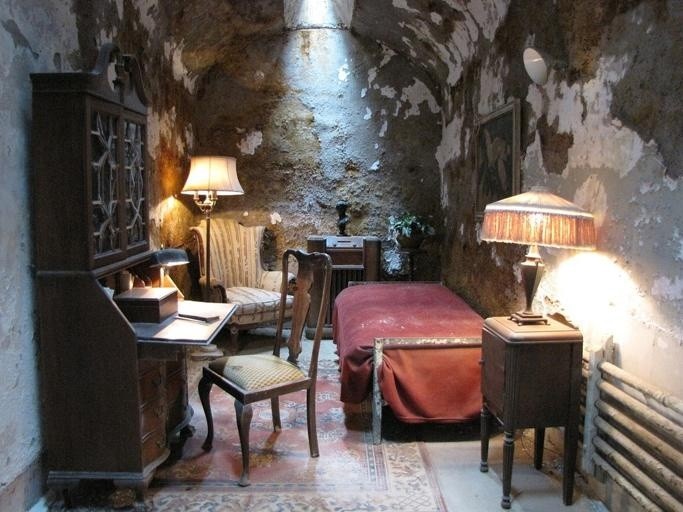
top-left (470, 97), bottom-right (522, 221)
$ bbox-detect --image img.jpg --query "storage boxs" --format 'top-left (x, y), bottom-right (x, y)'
top-left (115, 287), bottom-right (178, 324)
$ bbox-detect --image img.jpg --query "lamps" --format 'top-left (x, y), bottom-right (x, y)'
top-left (180, 155), bottom-right (246, 362)
top-left (148, 248), bottom-right (190, 288)
top-left (522, 46), bottom-right (560, 87)
top-left (478, 187), bottom-right (597, 326)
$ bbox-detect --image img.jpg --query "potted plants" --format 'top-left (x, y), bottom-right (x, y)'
top-left (389, 210), bottom-right (438, 249)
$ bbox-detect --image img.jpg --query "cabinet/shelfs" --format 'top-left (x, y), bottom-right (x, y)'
top-left (28, 43), bottom-right (240, 510)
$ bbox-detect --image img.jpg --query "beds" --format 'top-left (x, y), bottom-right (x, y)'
top-left (335, 279), bottom-right (486, 444)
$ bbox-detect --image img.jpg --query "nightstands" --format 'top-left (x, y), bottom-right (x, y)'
top-left (479, 316), bottom-right (583, 508)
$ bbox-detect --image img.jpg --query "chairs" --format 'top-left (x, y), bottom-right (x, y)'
top-left (188, 218), bottom-right (298, 356)
top-left (198, 248), bottom-right (331, 487)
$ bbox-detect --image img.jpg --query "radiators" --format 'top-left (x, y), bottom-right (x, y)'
top-left (577, 338), bottom-right (683, 512)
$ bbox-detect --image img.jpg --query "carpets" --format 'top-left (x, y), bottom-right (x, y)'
top-left (51, 360), bottom-right (449, 512)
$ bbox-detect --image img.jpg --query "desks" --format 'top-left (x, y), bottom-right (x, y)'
top-left (395, 251), bottom-right (426, 280)
top-left (305, 233), bottom-right (381, 340)
